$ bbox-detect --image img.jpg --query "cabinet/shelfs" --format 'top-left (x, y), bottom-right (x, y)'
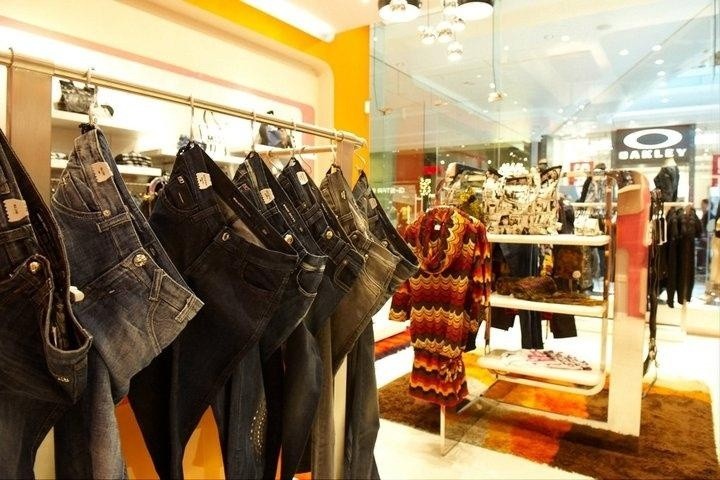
top-left (454, 226), bottom-right (611, 398)
top-left (51, 105), bottom-right (249, 181)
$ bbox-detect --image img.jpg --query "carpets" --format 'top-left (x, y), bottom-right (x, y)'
top-left (377, 367), bottom-right (720, 478)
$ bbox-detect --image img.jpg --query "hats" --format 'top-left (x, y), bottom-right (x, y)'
top-left (661, 158), bottom-right (677, 168)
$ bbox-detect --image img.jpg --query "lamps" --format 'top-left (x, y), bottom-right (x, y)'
top-left (361, 0), bottom-right (509, 119)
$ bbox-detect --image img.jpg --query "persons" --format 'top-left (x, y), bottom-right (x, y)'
top-left (695, 198), bottom-right (717, 275)
top-left (654, 156), bottom-right (681, 202)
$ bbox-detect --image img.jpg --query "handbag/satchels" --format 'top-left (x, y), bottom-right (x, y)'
top-left (57, 79), bottom-right (98, 115)
top-left (481, 165), bottom-right (563, 235)
top-left (259, 111), bottom-right (294, 148)
